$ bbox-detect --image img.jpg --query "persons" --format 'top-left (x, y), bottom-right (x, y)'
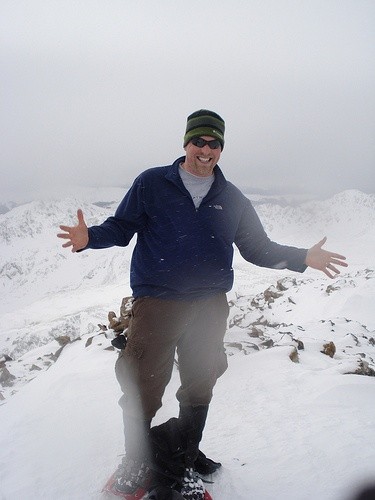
top-left (54, 108), bottom-right (348, 500)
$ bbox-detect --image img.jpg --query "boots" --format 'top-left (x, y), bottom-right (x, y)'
top-left (179, 405), bottom-right (218, 475)
top-left (115, 418), bottom-right (148, 492)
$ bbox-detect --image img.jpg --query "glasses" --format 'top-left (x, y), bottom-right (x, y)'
top-left (191, 137), bottom-right (220, 149)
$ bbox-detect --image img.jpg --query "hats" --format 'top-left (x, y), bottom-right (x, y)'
top-left (183, 110), bottom-right (225, 151)
